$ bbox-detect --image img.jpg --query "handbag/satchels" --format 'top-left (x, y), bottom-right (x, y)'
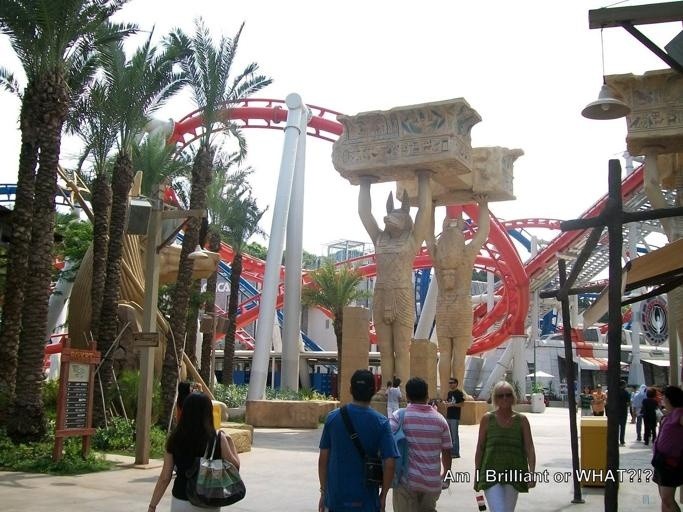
top-left (196, 429), bottom-right (245, 507)
top-left (650, 451), bottom-right (666, 471)
top-left (391, 410), bottom-right (409, 487)
top-left (340, 405), bottom-right (384, 487)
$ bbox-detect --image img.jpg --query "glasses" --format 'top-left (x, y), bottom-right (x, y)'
top-left (496, 393), bottom-right (512, 398)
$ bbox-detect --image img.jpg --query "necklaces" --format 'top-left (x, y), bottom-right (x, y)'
top-left (497, 411), bottom-right (512, 424)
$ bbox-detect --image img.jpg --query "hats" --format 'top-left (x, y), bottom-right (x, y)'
top-left (350, 369), bottom-right (375, 397)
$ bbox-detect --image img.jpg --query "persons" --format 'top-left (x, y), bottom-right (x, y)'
top-left (174, 380), bottom-right (204, 426)
top-left (439, 376), bottom-right (466, 458)
top-left (578, 378), bottom-right (671, 445)
top-left (146, 393), bottom-right (240, 511)
top-left (386, 378), bottom-right (403, 418)
top-left (650, 382), bottom-right (683, 511)
top-left (384, 376), bottom-right (453, 511)
top-left (316, 368), bottom-right (399, 512)
top-left (423, 193), bottom-right (492, 401)
top-left (354, 169), bottom-right (435, 394)
top-left (471, 381), bottom-right (537, 512)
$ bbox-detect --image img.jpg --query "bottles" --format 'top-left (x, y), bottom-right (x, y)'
top-left (475, 489), bottom-right (487, 511)
